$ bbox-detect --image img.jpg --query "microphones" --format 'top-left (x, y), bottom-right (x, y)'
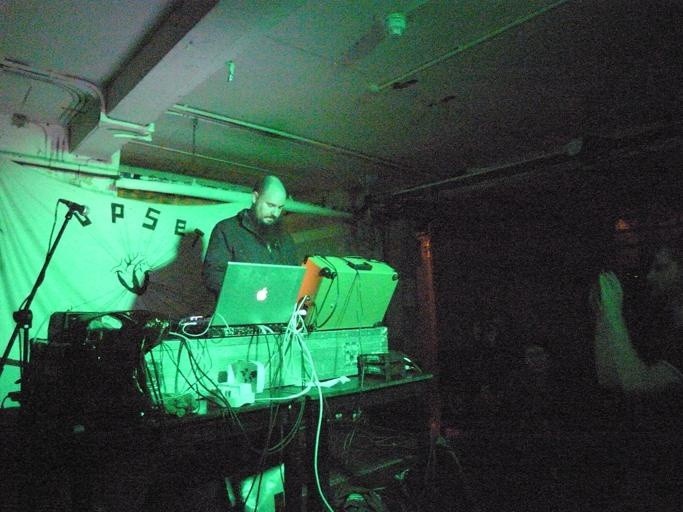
top-left (60, 198), bottom-right (90, 217)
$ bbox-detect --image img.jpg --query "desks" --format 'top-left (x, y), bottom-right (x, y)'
top-left (0, 368), bottom-right (440, 512)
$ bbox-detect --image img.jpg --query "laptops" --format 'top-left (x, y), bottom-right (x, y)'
top-left (208, 262), bottom-right (307, 325)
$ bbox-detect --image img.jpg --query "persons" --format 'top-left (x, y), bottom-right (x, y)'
top-left (199, 175), bottom-right (300, 307)
top-left (588, 242), bottom-right (681, 396)
